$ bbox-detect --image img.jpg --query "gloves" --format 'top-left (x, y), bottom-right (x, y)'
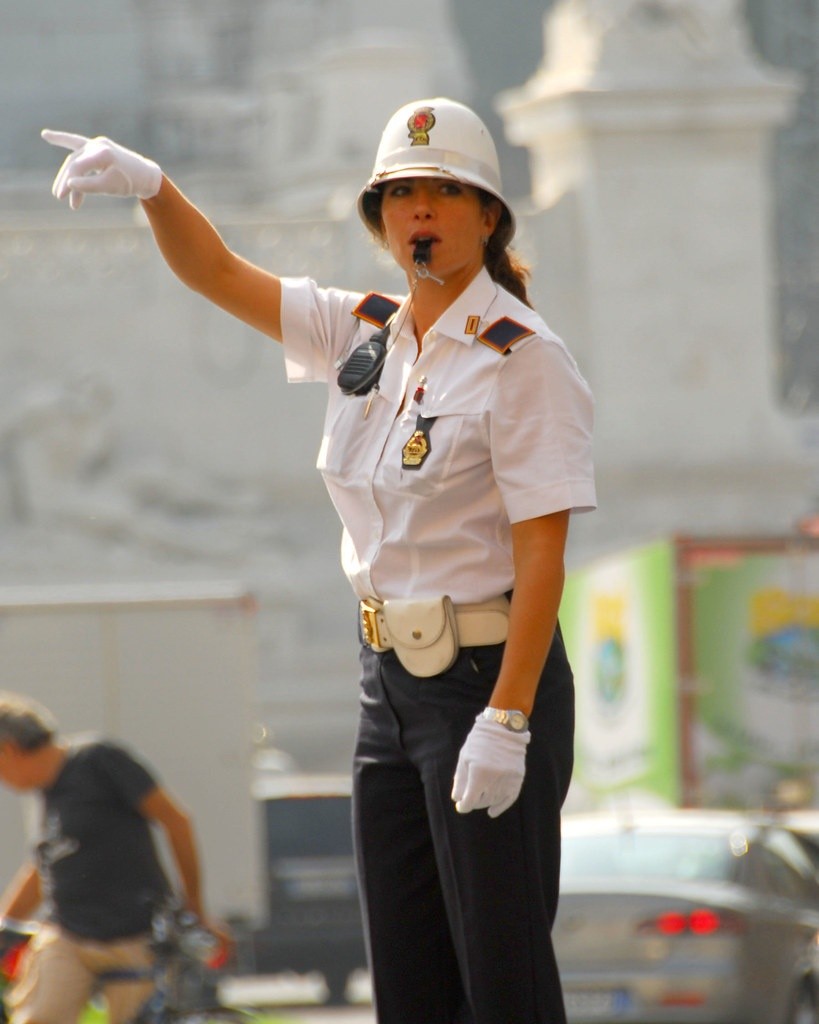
top-left (449, 717), bottom-right (532, 816)
top-left (40, 128), bottom-right (161, 211)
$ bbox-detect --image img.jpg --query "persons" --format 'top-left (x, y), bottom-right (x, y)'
top-left (40, 97), bottom-right (596, 1024)
top-left (0, 699), bottom-right (228, 1024)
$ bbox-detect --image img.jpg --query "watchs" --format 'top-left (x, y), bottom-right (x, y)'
top-left (482, 707), bottom-right (529, 733)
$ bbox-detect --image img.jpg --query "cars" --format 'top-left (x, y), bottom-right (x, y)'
top-left (550, 809), bottom-right (818, 1024)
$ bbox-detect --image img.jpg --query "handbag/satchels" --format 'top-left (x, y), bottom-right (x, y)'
top-left (377, 595), bottom-right (464, 682)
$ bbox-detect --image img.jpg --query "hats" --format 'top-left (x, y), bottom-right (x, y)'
top-left (356, 98), bottom-right (516, 253)
